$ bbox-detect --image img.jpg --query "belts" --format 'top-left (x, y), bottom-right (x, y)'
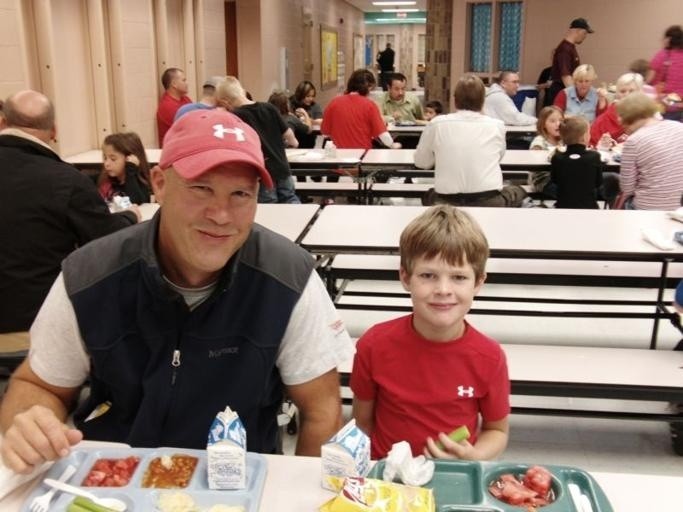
top-left (437, 190), bottom-right (499, 200)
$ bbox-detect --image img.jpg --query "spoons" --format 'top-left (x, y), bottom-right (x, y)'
top-left (43, 476), bottom-right (128, 510)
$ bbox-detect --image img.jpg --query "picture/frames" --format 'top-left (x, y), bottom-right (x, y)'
top-left (351, 32), bottom-right (363, 74)
top-left (318, 22), bottom-right (339, 93)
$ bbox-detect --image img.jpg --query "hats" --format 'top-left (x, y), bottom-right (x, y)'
top-left (203, 75), bottom-right (220, 90)
top-left (570, 18), bottom-right (593, 33)
top-left (160, 110), bottom-right (275, 191)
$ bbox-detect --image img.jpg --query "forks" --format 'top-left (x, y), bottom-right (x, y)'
top-left (29, 464), bottom-right (76, 511)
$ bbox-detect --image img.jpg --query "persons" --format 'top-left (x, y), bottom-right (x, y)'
top-left (0, 89), bottom-right (142, 381)
top-left (668, 280), bottom-right (683, 457)
top-left (96, 131), bottom-right (157, 206)
top-left (156, 18), bottom-right (683, 210)
top-left (2, 107), bottom-right (356, 477)
top-left (349, 204), bottom-right (511, 461)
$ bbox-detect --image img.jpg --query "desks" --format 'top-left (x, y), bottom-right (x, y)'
top-left (388, 125), bottom-right (538, 148)
top-left (308, 122), bottom-right (386, 131)
top-left (107, 198), bottom-right (319, 241)
top-left (303, 204), bottom-right (683, 348)
top-left (62, 146), bottom-right (364, 181)
top-left (362, 148), bottom-right (620, 184)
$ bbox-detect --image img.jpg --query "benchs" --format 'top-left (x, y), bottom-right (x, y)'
top-left (293, 180), bottom-right (359, 204)
top-left (331, 337), bottom-right (682, 452)
top-left (369, 182), bottom-right (534, 204)
